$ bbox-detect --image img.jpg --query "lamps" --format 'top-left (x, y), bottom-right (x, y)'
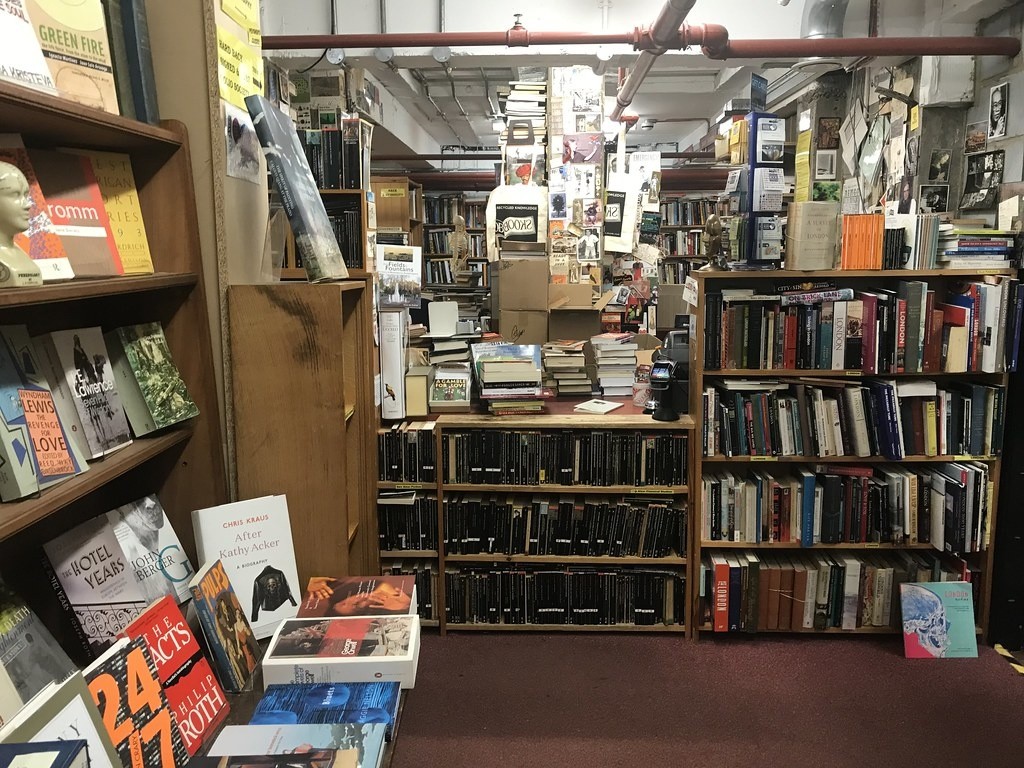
top-left (641, 120), bottom-right (654, 132)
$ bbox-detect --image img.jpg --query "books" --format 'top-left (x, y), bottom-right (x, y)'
top-left (0, 0), bottom-right (423, 768)
top-left (899, 580), bottom-right (979, 659)
top-left (300, 66), bottom-right (1024, 639)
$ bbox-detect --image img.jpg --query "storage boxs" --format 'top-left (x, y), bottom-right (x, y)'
top-left (601, 278), bottom-right (651, 332)
top-left (548, 309), bottom-right (601, 340)
top-left (489, 257), bottom-right (549, 311)
top-left (491, 310), bottom-right (548, 347)
top-left (548, 283), bottom-right (592, 310)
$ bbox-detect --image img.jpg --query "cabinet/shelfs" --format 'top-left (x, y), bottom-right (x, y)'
top-left (419, 192), bottom-right (737, 337)
top-left (370, 175), bottom-right (424, 287)
top-left (227, 280), bottom-right (365, 602)
top-left (382, 392), bottom-right (696, 644)
top-left (266, 184), bottom-right (375, 577)
top-left (685, 265), bottom-right (1020, 644)
top-left (0, 76), bottom-right (410, 768)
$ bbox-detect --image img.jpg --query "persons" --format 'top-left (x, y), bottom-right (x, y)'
top-left (991, 88), bottom-right (1006, 135)
top-left (0, 162), bottom-right (44, 287)
